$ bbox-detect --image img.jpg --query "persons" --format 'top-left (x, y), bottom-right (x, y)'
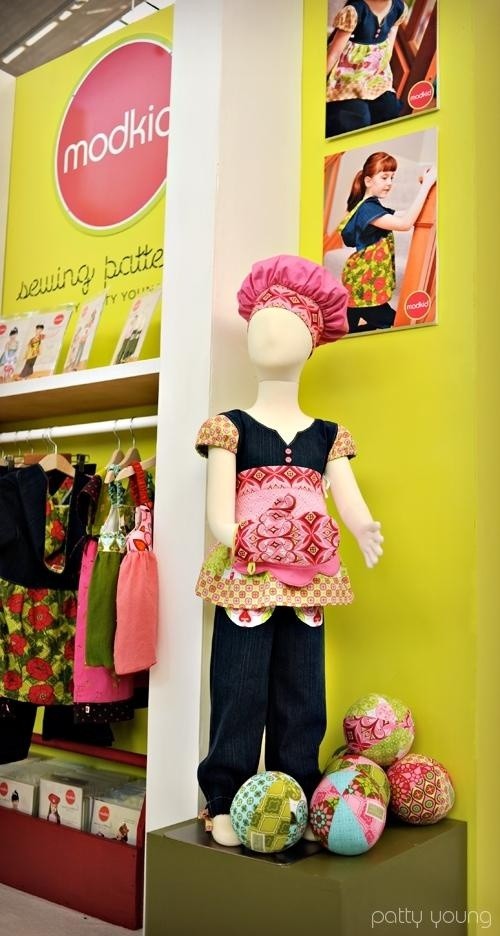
top-left (323, 146), bottom-right (438, 339)
top-left (323, 2), bottom-right (437, 142)
top-left (193, 250), bottom-right (389, 853)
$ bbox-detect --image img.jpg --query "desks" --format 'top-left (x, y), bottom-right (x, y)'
top-left (145, 812), bottom-right (469, 935)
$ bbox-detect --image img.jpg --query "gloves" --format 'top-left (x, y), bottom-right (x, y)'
top-left (231, 495), bottom-right (343, 587)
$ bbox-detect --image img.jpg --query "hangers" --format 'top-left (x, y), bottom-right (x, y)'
top-left (0, 424), bottom-right (90, 478)
top-left (104, 414), bottom-right (156, 485)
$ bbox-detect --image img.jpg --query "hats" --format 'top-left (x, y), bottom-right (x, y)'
top-left (234, 256), bottom-right (350, 358)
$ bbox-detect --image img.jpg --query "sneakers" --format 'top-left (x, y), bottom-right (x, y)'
top-left (199, 800), bottom-right (243, 847)
